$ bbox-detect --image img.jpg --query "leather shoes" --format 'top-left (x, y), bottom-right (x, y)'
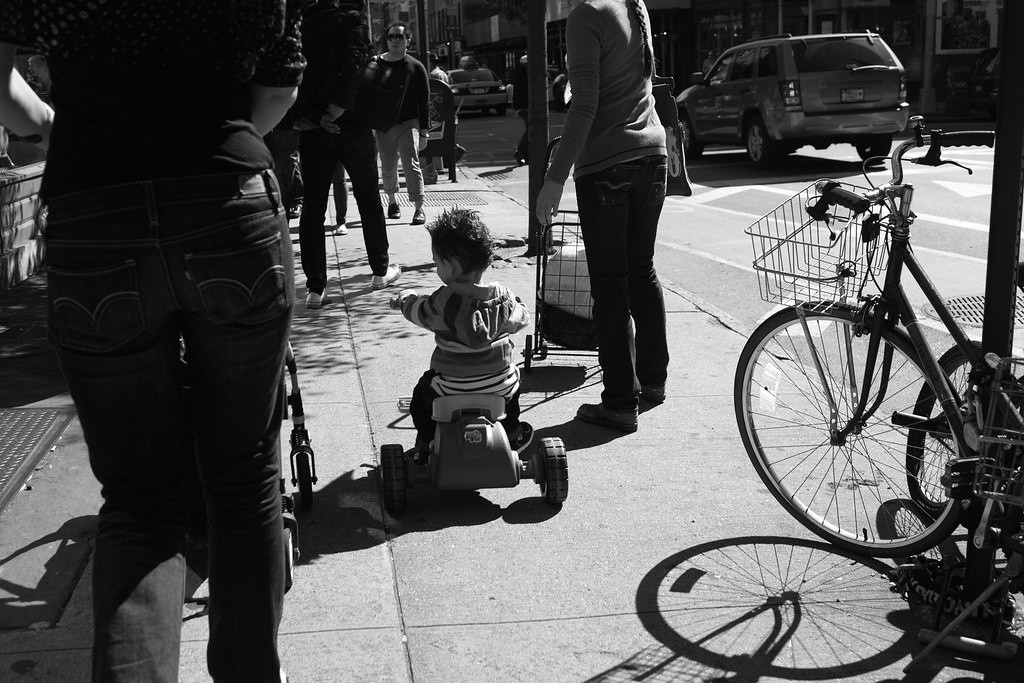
top-left (413, 209), bottom-right (425, 224)
top-left (388, 203), bottom-right (400, 218)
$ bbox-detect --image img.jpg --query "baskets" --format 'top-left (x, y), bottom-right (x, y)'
top-left (744, 177), bottom-right (886, 314)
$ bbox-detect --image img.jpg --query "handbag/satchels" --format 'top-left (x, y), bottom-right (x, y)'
top-left (651, 73), bottom-right (692, 195)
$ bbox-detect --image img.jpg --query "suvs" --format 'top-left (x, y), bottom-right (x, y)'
top-left (676, 29), bottom-right (909, 169)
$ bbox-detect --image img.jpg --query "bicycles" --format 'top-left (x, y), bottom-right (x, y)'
top-left (731, 116), bottom-right (1024, 558)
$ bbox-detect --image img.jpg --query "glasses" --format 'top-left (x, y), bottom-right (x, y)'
top-left (387, 33), bottom-right (410, 40)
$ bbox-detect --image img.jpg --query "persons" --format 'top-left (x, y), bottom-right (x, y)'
top-left (533, 0), bottom-right (670, 431)
top-left (388, 206), bottom-right (534, 457)
top-left (0, 0), bottom-right (308, 683)
top-left (261, 0), bottom-right (530, 310)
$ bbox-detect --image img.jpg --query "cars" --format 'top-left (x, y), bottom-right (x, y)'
top-left (446, 69), bottom-right (508, 116)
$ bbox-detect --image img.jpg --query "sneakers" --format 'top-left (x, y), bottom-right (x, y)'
top-left (640, 384), bottom-right (665, 400)
top-left (577, 401), bottom-right (639, 432)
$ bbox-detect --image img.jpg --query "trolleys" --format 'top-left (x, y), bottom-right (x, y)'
top-left (520, 136), bottom-right (596, 371)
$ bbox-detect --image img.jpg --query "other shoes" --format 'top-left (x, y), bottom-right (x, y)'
top-left (413, 449), bottom-right (430, 464)
top-left (337, 222), bottom-right (347, 233)
top-left (306, 287), bottom-right (327, 307)
top-left (372, 262), bottom-right (401, 287)
top-left (509, 421), bottom-right (534, 452)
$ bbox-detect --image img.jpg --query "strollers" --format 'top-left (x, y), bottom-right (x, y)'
top-left (174, 326), bottom-right (318, 590)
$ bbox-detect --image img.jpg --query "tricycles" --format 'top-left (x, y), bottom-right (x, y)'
top-left (380, 297), bottom-right (568, 512)
top-left (890, 261), bottom-right (1024, 519)
